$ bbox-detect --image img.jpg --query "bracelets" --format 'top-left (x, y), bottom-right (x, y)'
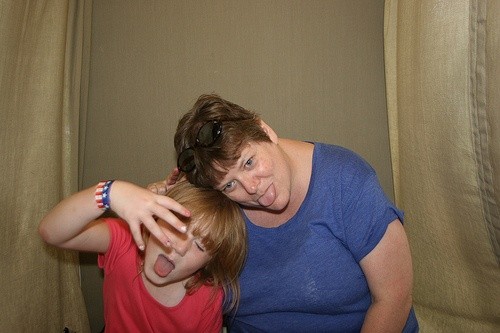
top-left (95, 180), bottom-right (114, 212)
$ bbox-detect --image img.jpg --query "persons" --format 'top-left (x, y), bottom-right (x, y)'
top-left (147, 94), bottom-right (419, 333)
top-left (39, 180), bottom-right (248, 332)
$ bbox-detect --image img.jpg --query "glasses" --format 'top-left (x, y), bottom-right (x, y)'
top-left (177, 116), bottom-right (252, 172)
